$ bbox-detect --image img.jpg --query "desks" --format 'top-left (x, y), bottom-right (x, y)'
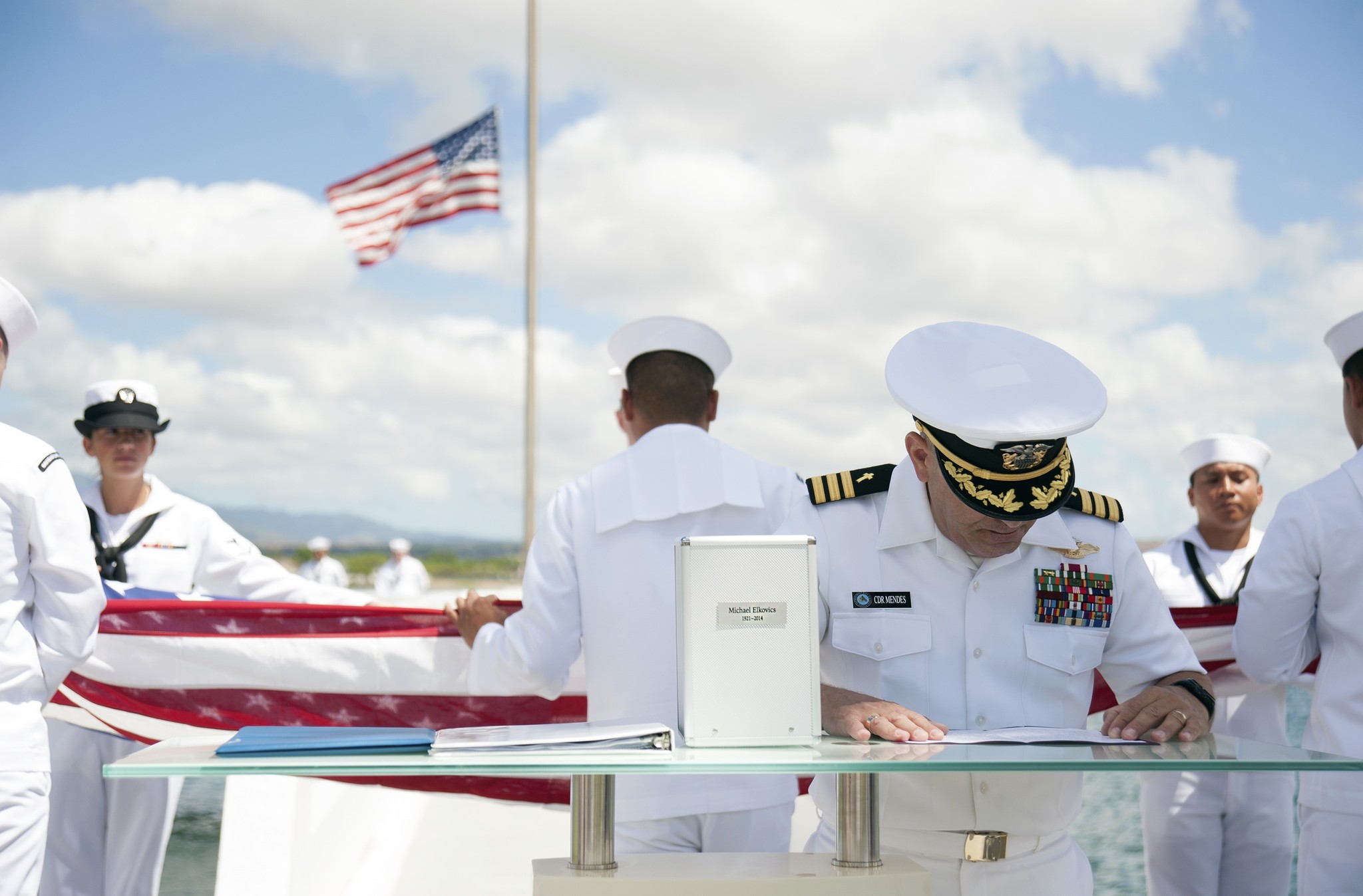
top-left (102, 728), bottom-right (1363, 895)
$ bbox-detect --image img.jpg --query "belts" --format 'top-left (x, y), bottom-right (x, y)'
top-left (822, 814), bottom-right (1068, 862)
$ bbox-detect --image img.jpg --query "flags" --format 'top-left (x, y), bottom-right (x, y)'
top-left (327, 108), bottom-right (500, 268)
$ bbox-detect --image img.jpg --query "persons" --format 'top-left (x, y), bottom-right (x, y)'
top-left (0, 276), bottom-right (108, 894)
top-left (296, 541), bottom-right (350, 590)
top-left (1233, 305), bottom-right (1363, 896)
top-left (38, 378), bottom-right (325, 896)
top-left (445, 314), bottom-right (829, 853)
top-left (804, 314), bottom-right (1220, 896)
top-left (1134, 432), bottom-right (1297, 896)
top-left (370, 538), bottom-right (430, 594)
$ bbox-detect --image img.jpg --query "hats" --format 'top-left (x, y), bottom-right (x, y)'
top-left (307, 537), bottom-right (332, 552)
top-left (1323, 312), bottom-right (1363, 369)
top-left (884, 321), bottom-right (1108, 521)
top-left (0, 277), bottom-right (39, 363)
top-left (389, 538), bottom-right (412, 552)
top-left (1173, 433), bottom-right (1273, 487)
top-left (75, 379), bottom-right (170, 431)
top-left (607, 314), bottom-right (732, 383)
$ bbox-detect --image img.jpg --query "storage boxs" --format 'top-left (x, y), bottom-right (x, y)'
top-left (673, 534), bottom-right (822, 750)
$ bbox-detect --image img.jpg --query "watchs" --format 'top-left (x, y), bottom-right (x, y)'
top-left (1168, 677), bottom-right (1217, 724)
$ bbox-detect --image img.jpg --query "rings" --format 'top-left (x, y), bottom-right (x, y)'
top-left (864, 713), bottom-right (880, 731)
top-left (1172, 709), bottom-right (1187, 723)
